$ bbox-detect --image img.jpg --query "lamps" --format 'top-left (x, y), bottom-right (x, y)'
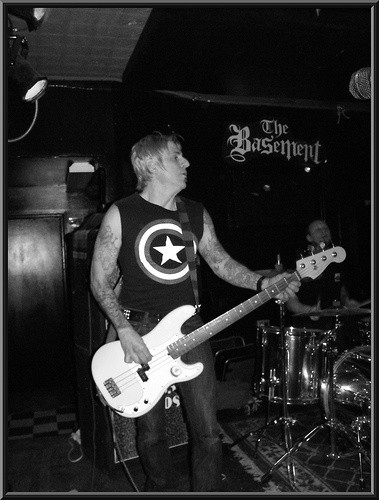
top-left (7, 6), bottom-right (50, 34)
top-left (5, 44), bottom-right (49, 104)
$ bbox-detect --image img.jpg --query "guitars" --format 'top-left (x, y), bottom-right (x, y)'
top-left (90, 240), bottom-right (347, 420)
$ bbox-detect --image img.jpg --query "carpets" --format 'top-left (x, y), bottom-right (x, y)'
top-left (219, 408), bottom-right (371, 493)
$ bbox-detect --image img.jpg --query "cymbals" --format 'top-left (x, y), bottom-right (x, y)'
top-left (292, 307), bottom-right (371, 317)
top-left (254, 269), bottom-right (323, 283)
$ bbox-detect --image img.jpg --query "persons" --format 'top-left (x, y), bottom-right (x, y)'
top-left (91, 131), bottom-right (301, 491)
top-left (286, 218), bottom-right (359, 329)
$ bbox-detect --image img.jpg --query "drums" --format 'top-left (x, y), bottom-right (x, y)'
top-left (249, 319), bottom-right (285, 408)
top-left (324, 345), bottom-right (371, 464)
top-left (260, 325), bottom-right (333, 405)
top-left (353, 316), bottom-right (371, 346)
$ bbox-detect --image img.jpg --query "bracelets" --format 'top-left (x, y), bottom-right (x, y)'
top-left (257, 276), bottom-right (265, 292)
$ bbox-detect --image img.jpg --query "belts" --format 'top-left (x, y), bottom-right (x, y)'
top-left (120, 308), bottom-right (165, 324)
